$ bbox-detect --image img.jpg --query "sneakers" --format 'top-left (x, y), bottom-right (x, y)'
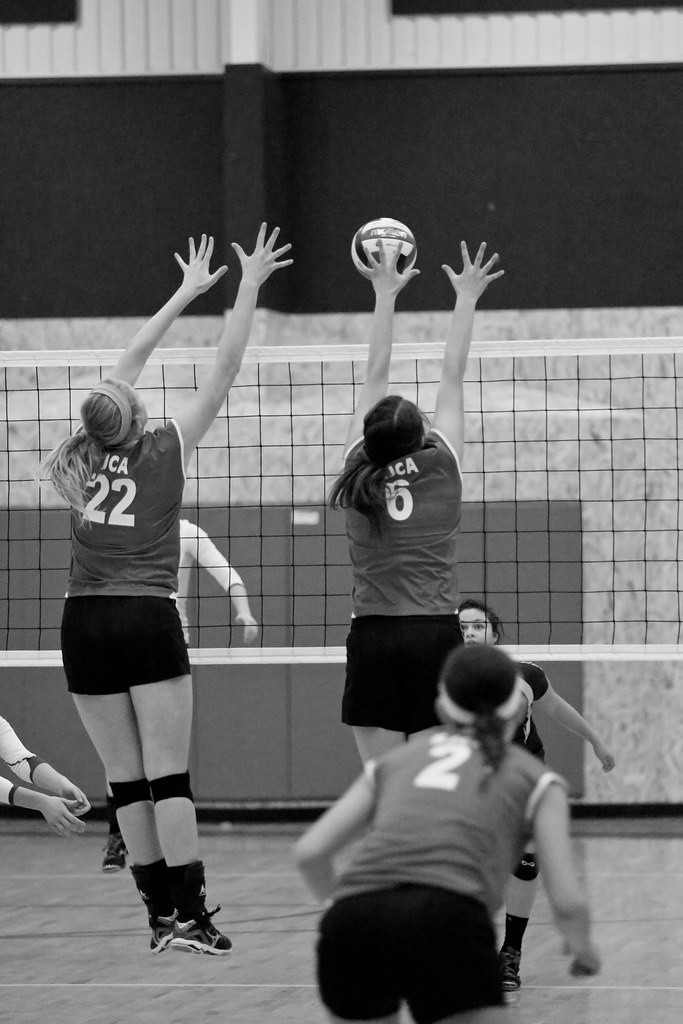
top-left (499, 946), bottom-right (522, 990)
top-left (170, 884), bottom-right (232, 956)
top-left (100, 833), bottom-right (128, 873)
top-left (136, 885), bottom-right (180, 955)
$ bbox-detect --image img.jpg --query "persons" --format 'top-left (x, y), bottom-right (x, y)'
top-left (41, 219), bottom-right (296, 959)
top-left (288, 644), bottom-right (601, 1024)
top-left (0, 715), bottom-right (92, 837)
top-left (329, 235), bottom-right (503, 769)
top-left (455, 594), bottom-right (619, 992)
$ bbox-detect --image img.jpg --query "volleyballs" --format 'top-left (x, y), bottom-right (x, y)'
top-left (349, 217), bottom-right (418, 280)
